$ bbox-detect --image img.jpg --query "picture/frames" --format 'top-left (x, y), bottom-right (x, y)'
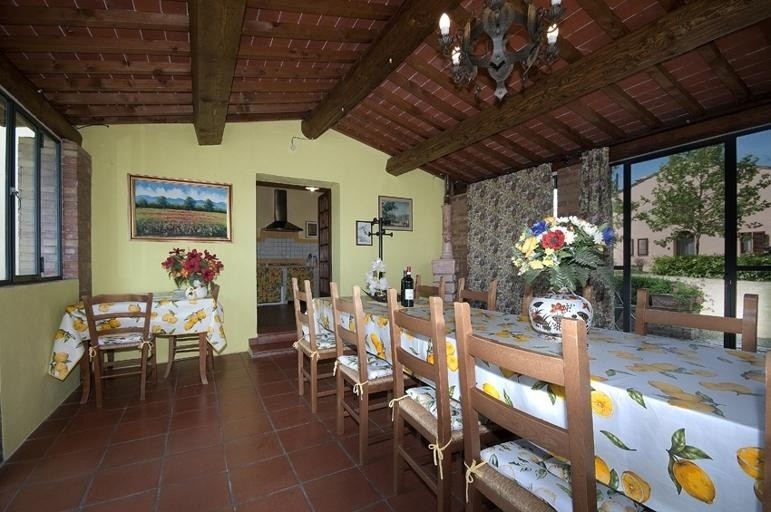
top-left (356, 220), bottom-right (373, 245)
top-left (305, 221), bottom-right (318, 238)
top-left (377, 195), bottom-right (413, 231)
top-left (127, 172), bottom-right (233, 242)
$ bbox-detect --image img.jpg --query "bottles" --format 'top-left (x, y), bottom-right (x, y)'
top-left (399, 265), bottom-right (416, 308)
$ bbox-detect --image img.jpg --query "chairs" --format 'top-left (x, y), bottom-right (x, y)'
top-left (81, 292), bottom-right (157, 408)
top-left (454, 301), bottom-right (645, 512)
top-left (385, 289), bottom-right (520, 510)
top-left (764, 350), bottom-right (771, 512)
top-left (636, 289), bottom-right (759, 352)
top-left (291, 278), bottom-right (422, 463)
top-left (412, 275), bottom-right (499, 312)
top-left (163, 283), bottom-right (220, 378)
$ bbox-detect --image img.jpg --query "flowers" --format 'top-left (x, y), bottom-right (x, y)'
top-left (511, 215), bottom-right (606, 294)
top-left (364, 257), bottom-right (390, 297)
top-left (161, 247), bottom-right (224, 287)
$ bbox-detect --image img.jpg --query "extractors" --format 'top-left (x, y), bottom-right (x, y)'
top-left (263, 188), bottom-right (304, 232)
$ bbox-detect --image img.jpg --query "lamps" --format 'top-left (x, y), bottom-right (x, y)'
top-left (436, 0), bottom-right (568, 102)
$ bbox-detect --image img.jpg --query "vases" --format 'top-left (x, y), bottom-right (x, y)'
top-left (529, 287), bottom-right (594, 344)
top-left (185, 280), bottom-right (208, 299)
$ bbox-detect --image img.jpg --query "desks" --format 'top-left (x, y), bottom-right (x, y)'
top-left (305, 294), bottom-right (764, 511)
